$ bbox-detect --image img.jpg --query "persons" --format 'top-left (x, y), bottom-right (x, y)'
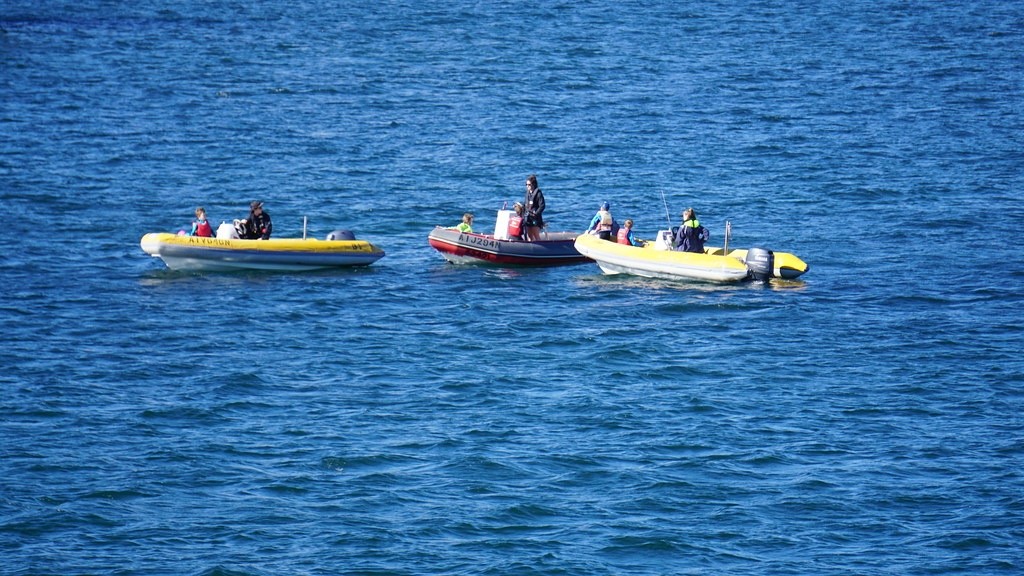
top-left (509, 202), bottom-right (528, 241)
top-left (525, 175), bottom-right (545, 241)
top-left (447, 213), bottom-right (474, 233)
top-left (190, 207), bottom-right (216, 238)
top-left (216, 200), bottom-right (273, 240)
top-left (675, 208), bottom-right (709, 253)
top-left (584, 200), bottom-right (613, 241)
top-left (617, 219), bottom-right (646, 247)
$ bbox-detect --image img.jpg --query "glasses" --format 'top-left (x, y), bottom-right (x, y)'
top-left (525, 184), bottom-right (533, 186)
top-left (253, 207), bottom-right (262, 209)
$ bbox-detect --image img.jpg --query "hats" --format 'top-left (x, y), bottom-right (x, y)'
top-left (250, 201), bottom-right (264, 208)
top-left (601, 201), bottom-right (610, 208)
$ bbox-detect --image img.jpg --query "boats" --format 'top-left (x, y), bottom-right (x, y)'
top-left (648, 241), bottom-right (811, 278)
top-left (428, 225), bottom-right (629, 265)
top-left (574, 229), bottom-right (754, 285)
top-left (141, 233), bottom-right (387, 269)
